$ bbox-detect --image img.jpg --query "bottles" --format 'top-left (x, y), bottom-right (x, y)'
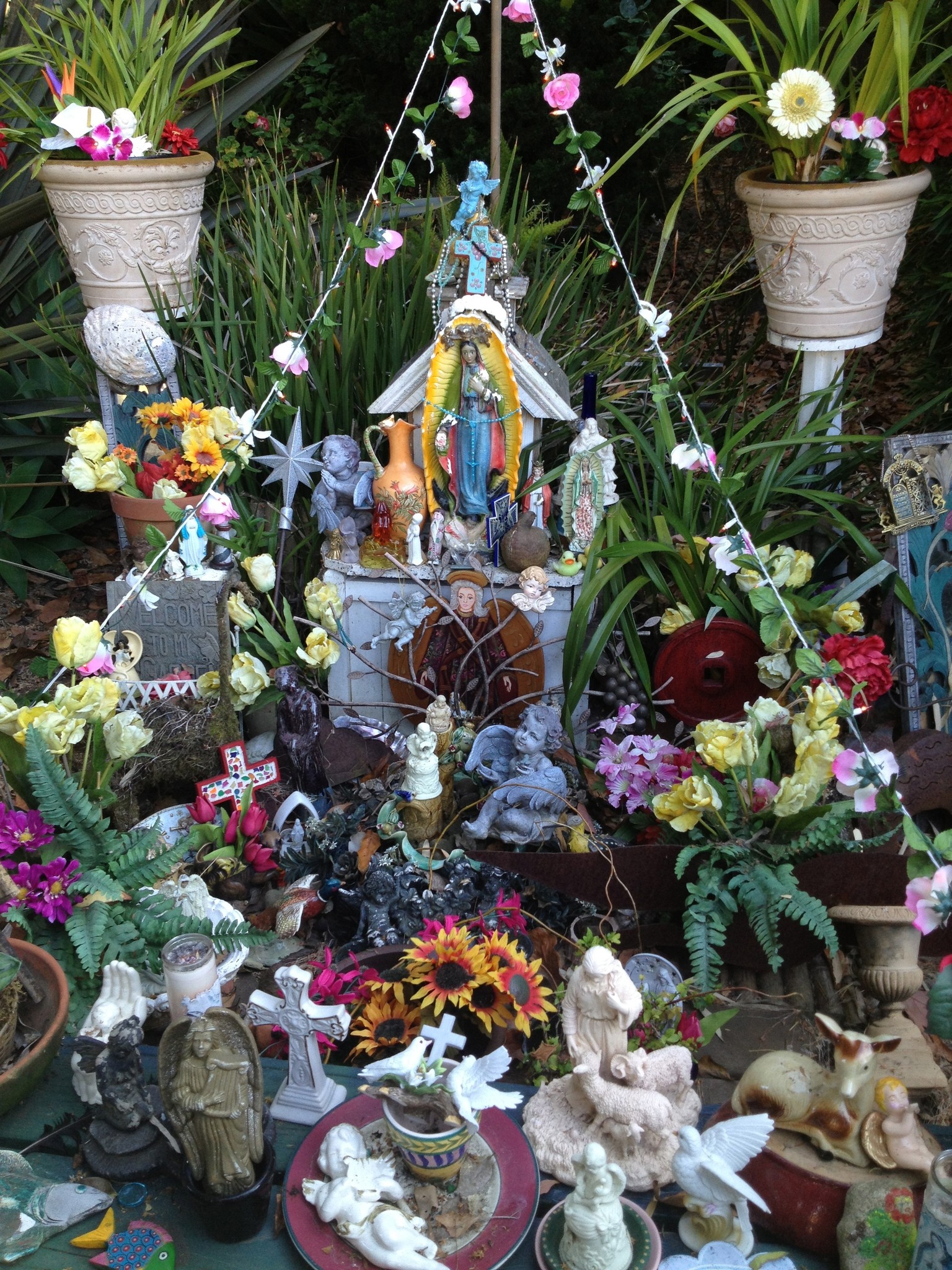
top-left (363, 414), bottom-right (429, 555)
top-left (160, 933), bottom-right (222, 1021)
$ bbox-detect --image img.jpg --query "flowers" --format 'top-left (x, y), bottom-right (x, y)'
top-left (250, 890), bottom-right (557, 1066)
top-left (0, 722), bottom-right (279, 981)
top-left (0, 0), bottom-right (481, 874)
top-left (591, 427), bottom-right (952, 996)
top-left (589, 1), bottom-right (952, 343)
top-left (503, 0), bottom-right (580, 111)
top-left (523, 927), bottom-right (739, 1089)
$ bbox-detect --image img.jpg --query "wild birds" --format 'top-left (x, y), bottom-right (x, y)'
top-left (670, 1114), bottom-right (777, 1220)
top-left (445, 1043), bottom-right (525, 1128)
top-left (358, 1033), bottom-right (433, 1090)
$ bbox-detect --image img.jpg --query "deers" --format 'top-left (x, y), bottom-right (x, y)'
top-left (731, 1008), bottom-right (903, 1168)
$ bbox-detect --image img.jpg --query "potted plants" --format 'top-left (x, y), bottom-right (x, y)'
top-left (0, 0), bottom-right (258, 311)
top-left (589, 0), bottom-right (952, 338)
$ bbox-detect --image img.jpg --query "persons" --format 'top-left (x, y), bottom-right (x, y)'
top-left (372, 592), bottom-right (438, 651)
top-left (71, 163), bottom-right (933, 1270)
top-left (462, 704), bottom-right (566, 843)
top-left (425, 695), bottom-right (450, 732)
top-left (309, 435), bottom-right (377, 546)
top-left (570, 455), bottom-right (598, 539)
top-left (407, 723), bottom-right (437, 759)
top-left (511, 566), bottom-right (554, 613)
top-left (457, 340), bottom-right (503, 517)
top-left (860, 1077), bottom-right (934, 1174)
top-left (520, 460), bottom-right (551, 529)
top-left (353, 872), bottom-right (400, 947)
top-left (173, 1018), bottom-right (253, 1195)
top-left (417, 571), bottom-right (515, 729)
top-left (561, 945), bottom-right (642, 1114)
